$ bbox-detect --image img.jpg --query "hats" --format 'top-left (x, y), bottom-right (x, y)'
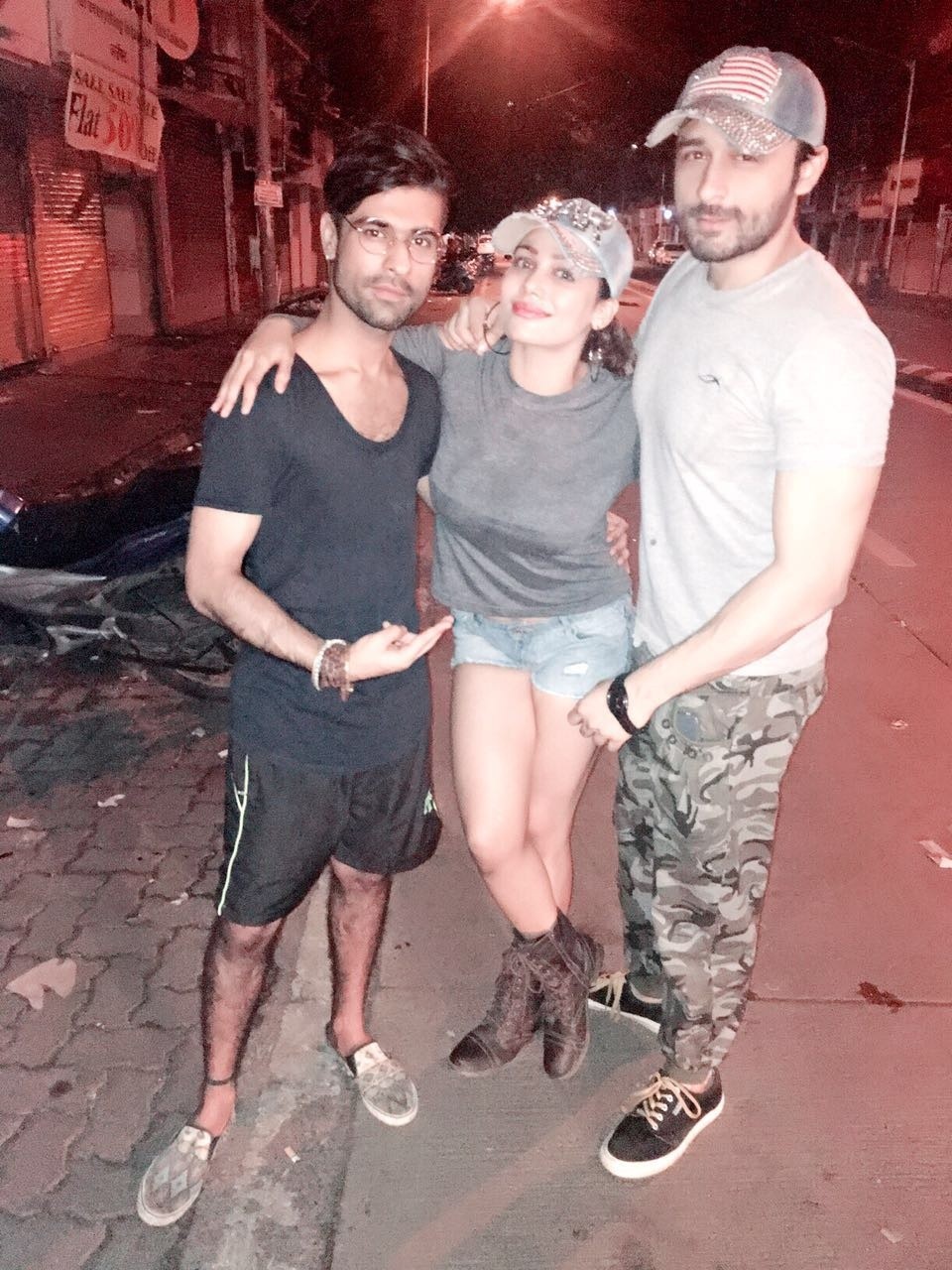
top-left (492, 197), bottom-right (636, 299)
top-left (645, 44), bottom-right (827, 155)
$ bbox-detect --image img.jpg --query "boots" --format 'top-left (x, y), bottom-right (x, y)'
top-left (446, 928), bottom-right (547, 1078)
top-left (513, 905), bottom-right (606, 1082)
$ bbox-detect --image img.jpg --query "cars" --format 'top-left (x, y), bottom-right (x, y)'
top-left (649, 239), bottom-right (686, 269)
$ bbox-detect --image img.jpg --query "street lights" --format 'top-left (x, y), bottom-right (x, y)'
top-left (830, 29), bottom-right (919, 283)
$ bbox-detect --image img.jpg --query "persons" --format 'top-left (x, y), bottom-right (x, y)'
top-left (437, 42), bottom-right (902, 1182)
top-left (112, 133), bottom-right (635, 1231)
top-left (204, 195), bottom-right (653, 1058)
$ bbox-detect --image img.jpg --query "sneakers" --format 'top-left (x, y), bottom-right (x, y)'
top-left (587, 970), bottom-right (665, 1034)
top-left (599, 1066), bottom-right (725, 1179)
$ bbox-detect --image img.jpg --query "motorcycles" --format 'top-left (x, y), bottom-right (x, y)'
top-left (0, 466), bottom-right (247, 700)
top-left (433, 231), bottom-right (491, 293)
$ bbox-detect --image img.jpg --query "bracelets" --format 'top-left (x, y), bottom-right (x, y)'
top-left (308, 635), bottom-right (356, 700)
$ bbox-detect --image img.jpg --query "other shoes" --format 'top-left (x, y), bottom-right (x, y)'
top-left (136, 1104), bottom-right (236, 1227)
top-left (324, 1021), bottom-right (419, 1127)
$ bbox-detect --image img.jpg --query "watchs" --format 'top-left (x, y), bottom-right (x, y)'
top-left (604, 669), bottom-right (653, 737)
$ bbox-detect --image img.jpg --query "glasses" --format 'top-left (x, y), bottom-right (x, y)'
top-left (338, 211), bottom-right (445, 265)
